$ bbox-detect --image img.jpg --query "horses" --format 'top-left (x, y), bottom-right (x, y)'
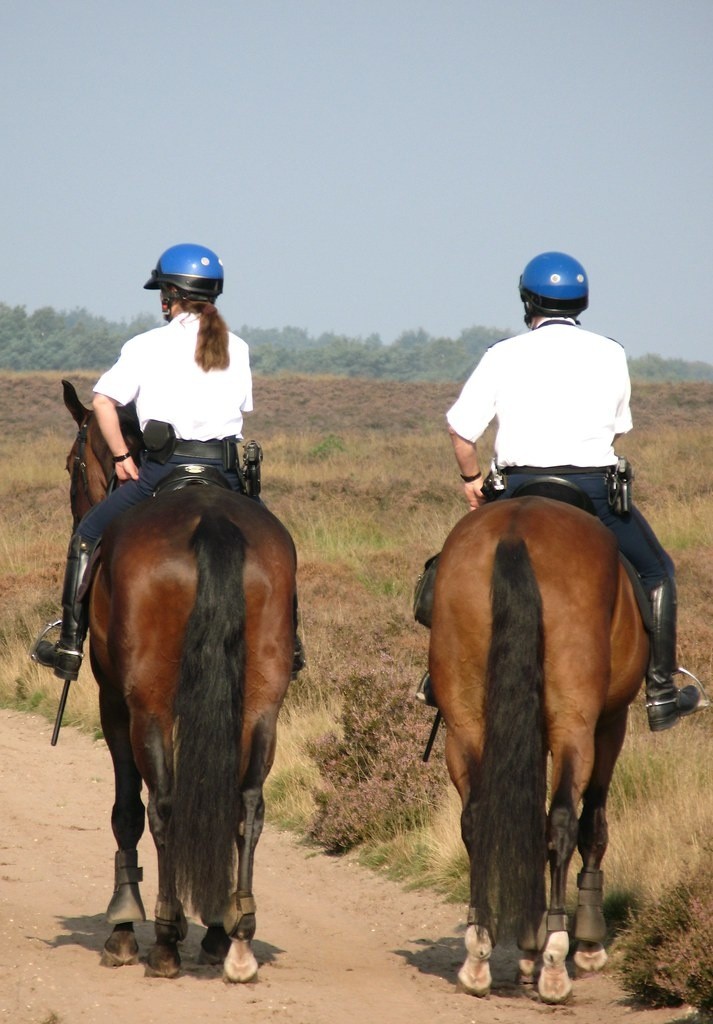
top-left (59, 373), bottom-right (300, 986)
top-left (427, 491), bottom-right (652, 1001)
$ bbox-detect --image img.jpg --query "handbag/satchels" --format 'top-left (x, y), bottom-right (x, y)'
top-left (413, 551), bottom-right (439, 628)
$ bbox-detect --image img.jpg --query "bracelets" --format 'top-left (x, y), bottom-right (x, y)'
top-left (112, 452), bottom-right (131, 462)
top-left (460, 472), bottom-right (481, 481)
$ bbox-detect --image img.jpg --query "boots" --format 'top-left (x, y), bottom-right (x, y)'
top-left (33, 534), bottom-right (103, 681)
top-left (644, 578), bottom-right (702, 732)
top-left (415, 671), bottom-right (436, 708)
top-left (288, 595), bottom-right (305, 680)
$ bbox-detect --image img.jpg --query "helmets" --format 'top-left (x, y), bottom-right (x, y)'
top-left (143, 243), bottom-right (225, 293)
top-left (518, 251), bottom-right (590, 314)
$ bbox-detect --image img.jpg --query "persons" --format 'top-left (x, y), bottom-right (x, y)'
top-left (30, 244), bottom-right (307, 680)
top-left (417, 252), bottom-right (700, 731)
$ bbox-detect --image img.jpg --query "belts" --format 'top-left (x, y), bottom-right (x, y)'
top-left (172, 441), bottom-right (225, 460)
top-left (499, 464), bottom-right (616, 475)
